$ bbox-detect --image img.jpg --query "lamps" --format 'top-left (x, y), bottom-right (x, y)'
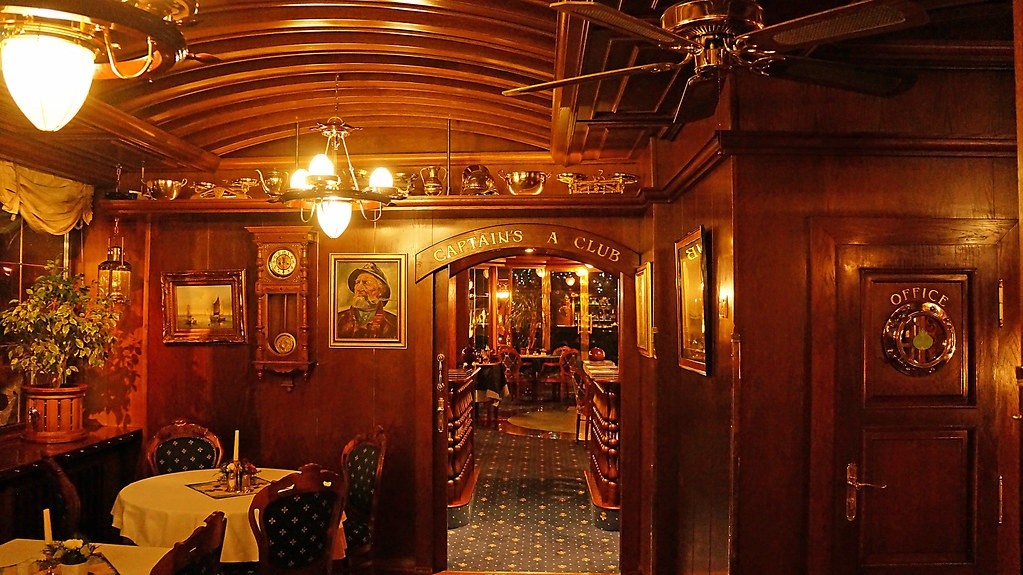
top-left (255, 72), bottom-right (409, 242)
top-left (0, 0), bottom-right (224, 134)
top-left (96, 217), bottom-right (131, 307)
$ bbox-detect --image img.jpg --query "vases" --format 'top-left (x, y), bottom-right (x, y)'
top-left (228, 473), bottom-right (251, 489)
top-left (54, 560), bottom-right (89, 575)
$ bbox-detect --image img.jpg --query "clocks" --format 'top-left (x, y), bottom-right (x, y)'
top-left (245, 225), bottom-right (315, 383)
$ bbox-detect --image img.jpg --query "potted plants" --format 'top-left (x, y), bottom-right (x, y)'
top-left (0, 249), bottom-right (123, 445)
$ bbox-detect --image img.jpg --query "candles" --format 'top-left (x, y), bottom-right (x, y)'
top-left (233, 430), bottom-right (239, 461)
top-left (43, 509), bottom-right (52, 544)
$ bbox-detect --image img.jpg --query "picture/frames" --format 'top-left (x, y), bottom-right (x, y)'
top-left (634, 263), bottom-right (654, 360)
top-left (673, 225), bottom-right (716, 378)
top-left (160, 267), bottom-right (250, 347)
top-left (327, 252), bottom-right (409, 351)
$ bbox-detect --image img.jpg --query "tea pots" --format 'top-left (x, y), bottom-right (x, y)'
top-left (255, 167), bottom-right (289, 201)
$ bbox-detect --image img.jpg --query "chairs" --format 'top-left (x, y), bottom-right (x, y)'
top-left (498, 348), bottom-right (532, 402)
top-left (248, 462), bottom-right (346, 575)
top-left (144, 417), bottom-right (224, 477)
top-left (552, 346), bottom-right (571, 356)
top-left (538, 348), bottom-right (581, 404)
top-left (331, 423), bottom-right (387, 575)
top-left (149, 511), bottom-right (227, 575)
top-left (474, 362), bottom-right (507, 430)
top-left (568, 364), bottom-right (591, 449)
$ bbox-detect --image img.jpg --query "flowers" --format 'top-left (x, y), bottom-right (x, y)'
top-left (31, 535), bottom-right (102, 570)
top-left (212, 458), bottom-right (262, 482)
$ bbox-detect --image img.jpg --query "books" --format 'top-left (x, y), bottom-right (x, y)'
top-left (582, 360), bottom-right (619, 379)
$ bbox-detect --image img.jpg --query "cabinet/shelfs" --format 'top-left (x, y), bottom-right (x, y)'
top-left (465, 257), bottom-right (619, 361)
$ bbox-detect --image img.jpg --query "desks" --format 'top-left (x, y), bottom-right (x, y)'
top-left (0, 538), bottom-right (173, 575)
top-left (520, 355), bottom-right (562, 397)
top-left (0, 423), bottom-right (144, 543)
top-left (110, 467), bottom-right (348, 564)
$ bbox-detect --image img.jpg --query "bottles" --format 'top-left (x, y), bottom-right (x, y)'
top-left (526, 348), bottom-right (529, 356)
top-left (477, 354), bottom-right (482, 362)
top-left (464, 365), bottom-right (473, 377)
top-left (533, 347), bottom-right (547, 356)
top-left (420, 166), bottom-right (447, 196)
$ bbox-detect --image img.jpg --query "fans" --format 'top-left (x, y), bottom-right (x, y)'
top-left (503, 0), bottom-right (923, 125)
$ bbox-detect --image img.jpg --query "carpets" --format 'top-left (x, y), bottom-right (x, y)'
top-left (476, 404), bottom-right (591, 441)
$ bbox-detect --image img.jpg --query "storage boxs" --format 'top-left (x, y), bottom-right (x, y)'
top-left (568, 176), bottom-right (625, 194)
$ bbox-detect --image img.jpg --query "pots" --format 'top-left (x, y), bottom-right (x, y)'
top-left (498, 169), bottom-right (554, 196)
top-left (141, 178), bottom-right (187, 200)
